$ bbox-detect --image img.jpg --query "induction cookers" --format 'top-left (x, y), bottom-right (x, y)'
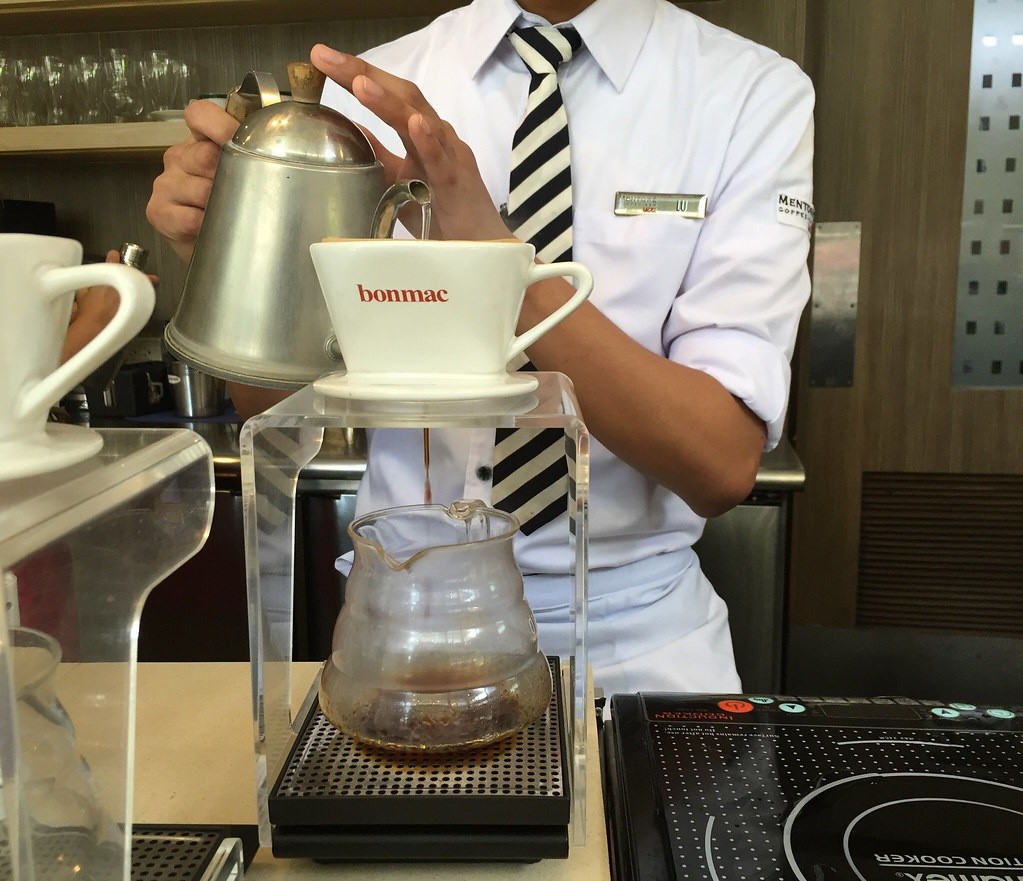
top-left (602, 691), bottom-right (1023, 881)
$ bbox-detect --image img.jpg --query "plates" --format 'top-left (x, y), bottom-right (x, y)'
top-left (149, 109), bottom-right (187, 120)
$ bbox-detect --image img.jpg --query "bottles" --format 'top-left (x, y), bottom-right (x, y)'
top-left (90, 242), bottom-right (148, 391)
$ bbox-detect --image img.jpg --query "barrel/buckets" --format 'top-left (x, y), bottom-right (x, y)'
top-left (165, 361), bottom-right (226, 417)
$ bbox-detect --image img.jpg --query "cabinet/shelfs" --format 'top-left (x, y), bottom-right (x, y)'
top-left (138, 488), bottom-right (357, 662)
top-left (0, 0), bottom-right (818, 416)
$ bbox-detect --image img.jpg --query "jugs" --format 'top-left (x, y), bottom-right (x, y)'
top-left (0, 625), bottom-right (131, 881)
top-left (318, 499), bottom-right (552, 760)
top-left (160, 62), bottom-right (431, 391)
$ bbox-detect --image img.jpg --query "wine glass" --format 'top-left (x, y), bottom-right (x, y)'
top-left (72, 55), bottom-right (108, 123)
top-left (102, 46), bottom-right (145, 122)
top-left (1, 55), bottom-right (72, 126)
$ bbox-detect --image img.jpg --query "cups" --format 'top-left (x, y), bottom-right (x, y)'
top-left (189, 93), bottom-right (227, 109)
top-left (139, 49), bottom-right (201, 119)
top-left (0, 232), bottom-right (155, 484)
top-left (308, 241), bottom-right (594, 400)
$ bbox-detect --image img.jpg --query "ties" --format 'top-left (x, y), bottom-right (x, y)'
top-left (490, 25), bottom-right (583, 537)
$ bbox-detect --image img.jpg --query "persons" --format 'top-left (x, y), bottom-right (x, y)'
top-left (146, 0), bottom-right (815, 725)
top-left (11, 247), bottom-right (159, 661)
